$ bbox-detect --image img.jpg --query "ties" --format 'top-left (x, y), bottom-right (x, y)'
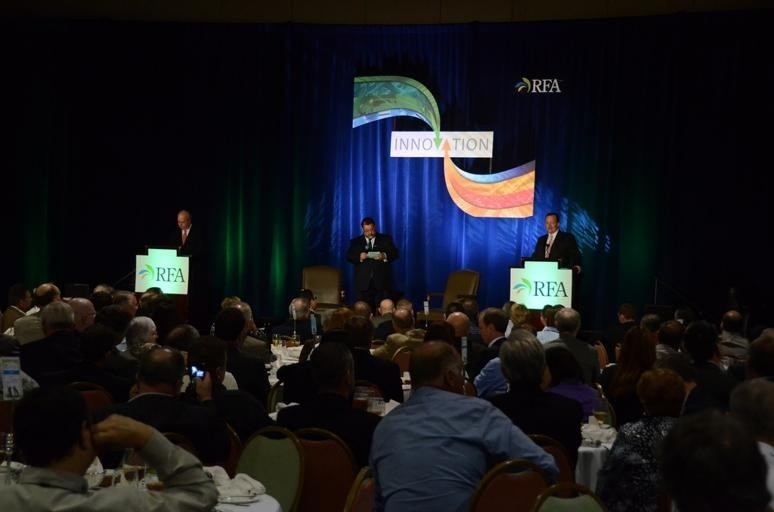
top-left (181, 230), bottom-right (189, 245)
top-left (366, 239), bottom-right (374, 252)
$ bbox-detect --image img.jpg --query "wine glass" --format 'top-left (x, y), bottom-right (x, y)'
top-left (592, 397), bottom-right (610, 425)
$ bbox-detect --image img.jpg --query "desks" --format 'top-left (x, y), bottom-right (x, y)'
top-left (266, 340), bottom-right (320, 412)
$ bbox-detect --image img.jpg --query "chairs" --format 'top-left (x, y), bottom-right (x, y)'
top-left (1, 303), bottom-right (45, 343)
top-left (392, 326), bottom-right (426, 390)
top-left (471, 425), bottom-right (617, 512)
top-left (596, 339), bottom-right (622, 424)
top-left (208, 418), bottom-right (377, 511)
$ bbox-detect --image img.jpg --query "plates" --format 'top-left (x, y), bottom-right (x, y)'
top-left (215, 493), bottom-right (260, 505)
top-left (0, 460), bottom-right (26, 475)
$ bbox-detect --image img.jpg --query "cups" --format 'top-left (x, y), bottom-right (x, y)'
top-left (269, 332), bottom-right (302, 368)
top-left (120, 447), bottom-right (151, 484)
top-left (353, 386), bottom-right (385, 416)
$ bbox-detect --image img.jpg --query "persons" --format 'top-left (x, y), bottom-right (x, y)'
top-left (172, 210), bottom-right (203, 257)
top-left (1, 280), bottom-right (773, 511)
top-left (347, 214), bottom-right (396, 301)
top-left (522, 212), bottom-right (584, 279)
